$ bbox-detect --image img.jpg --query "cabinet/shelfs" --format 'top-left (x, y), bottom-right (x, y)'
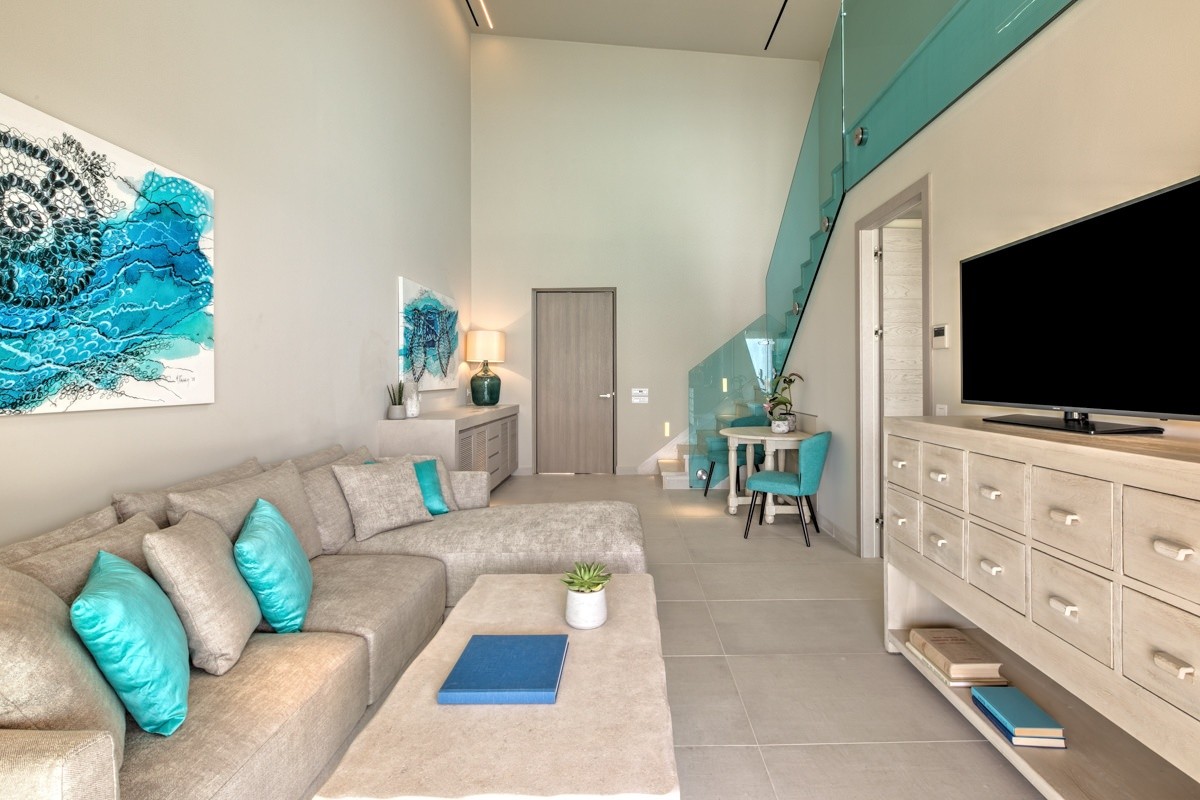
top-left (884, 412), bottom-right (1200, 800)
top-left (380, 403), bottom-right (520, 490)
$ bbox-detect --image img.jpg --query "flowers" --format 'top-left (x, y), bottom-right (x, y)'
top-left (763, 400), bottom-right (787, 419)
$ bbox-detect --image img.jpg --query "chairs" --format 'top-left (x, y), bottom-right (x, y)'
top-left (744, 430), bottom-right (831, 546)
top-left (703, 417), bottom-right (770, 497)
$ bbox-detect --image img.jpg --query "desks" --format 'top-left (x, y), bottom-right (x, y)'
top-left (719, 426), bottom-right (812, 525)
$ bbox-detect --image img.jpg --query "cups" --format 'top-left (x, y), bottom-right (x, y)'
top-left (403, 382), bottom-right (420, 417)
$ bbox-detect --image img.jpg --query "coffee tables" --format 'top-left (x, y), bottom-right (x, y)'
top-left (315, 574), bottom-right (680, 800)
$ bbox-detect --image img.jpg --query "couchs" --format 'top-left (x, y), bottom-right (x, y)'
top-left (269, 445), bottom-right (647, 623)
top-left (0, 458), bottom-right (447, 800)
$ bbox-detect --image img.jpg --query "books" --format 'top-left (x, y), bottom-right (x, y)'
top-left (909, 628), bottom-right (1003, 679)
top-left (971, 685), bottom-right (1065, 738)
top-left (906, 640), bottom-right (1008, 688)
top-left (971, 694), bottom-right (1067, 749)
top-left (438, 633), bottom-right (570, 705)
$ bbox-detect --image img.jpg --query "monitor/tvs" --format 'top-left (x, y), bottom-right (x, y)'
top-left (961, 175), bottom-right (1200, 434)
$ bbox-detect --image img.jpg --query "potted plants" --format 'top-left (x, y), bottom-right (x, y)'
top-left (387, 380), bottom-right (406, 420)
top-left (560, 561), bottom-right (613, 631)
top-left (770, 416), bottom-right (789, 433)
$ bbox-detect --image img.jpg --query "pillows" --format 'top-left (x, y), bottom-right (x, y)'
top-left (0, 452), bottom-right (459, 770)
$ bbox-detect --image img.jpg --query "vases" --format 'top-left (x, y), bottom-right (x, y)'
top-left (780, 412), bottom-right (797, 431)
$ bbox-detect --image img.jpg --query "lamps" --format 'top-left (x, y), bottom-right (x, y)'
top-left (466, 330), bottom-right (505, 406)
